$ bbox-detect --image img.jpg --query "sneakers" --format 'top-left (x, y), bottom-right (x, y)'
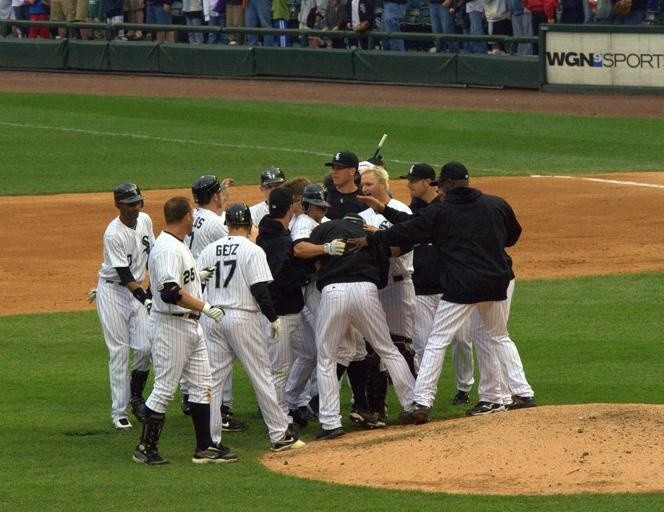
top-left (128, 391), bottom-right (148, 424)
top-left (398, 402), bottom-right (434, 424)
top-left (181, 393), bottom-right (192, 416)
top-left (270, 428), bottom-right (302, 452)
top-left (113, 417), bottom-right (133, 431)
top-left (509, 395), bottom-right (537, 408)
top-left (314, 424), bottom-right (345, 441)
top-left (221, 413), bottom-right (249, 432)
top-left (286, 393), bottom-right (320, 429)
top-left (466, 400), bottom-right (510, 416)
top-left (348, 392), bottom-right (389, 429)
top-left (191, 443), bottom-right (239, 464)
top-left (452, 389), bottom-right (470, 404)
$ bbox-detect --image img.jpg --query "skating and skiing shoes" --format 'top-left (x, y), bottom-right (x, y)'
top-left (132, 442), bottom-right (170, 465)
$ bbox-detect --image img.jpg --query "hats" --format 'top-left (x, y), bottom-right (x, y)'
top-left (324, 150), bottom-right (359, 171)
top-left (357, 155), bottom-right (384, 176)
top-left (429, 162), bottom-right (469, 186)
top-left (223, 201), bottom-right (254, 226)
top-left (399, 163), bottom-right (436, 181)
top-left (300, 181), bottom-right (334, 209)
top-left (266, 184), bottom-right (296, 218)
top-left (260, 166), bottom-right (287, 188)
top-left (189, 174), bottom-right (228, 204)
top-left (113, 180), bottom-right (148, 205)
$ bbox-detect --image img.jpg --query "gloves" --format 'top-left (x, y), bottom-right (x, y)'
top-left (143, 298), bottom-right (152, 314)
top-left (267, 317), bottom-right (282, 344)
top-left (198, 265), bottom-right (217, 283)
top-left (323, 235), bottom-right (349, 258)
top-left (207, 305), bottom-right (226, 323)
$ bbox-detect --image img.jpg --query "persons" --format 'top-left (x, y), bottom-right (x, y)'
top-left (95, 151), bottom-right (536, 466)
top-left (0, 0), bottom-right (664, 56)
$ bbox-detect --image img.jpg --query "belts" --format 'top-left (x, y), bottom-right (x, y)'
top-left (392, 271), bottom-right (415, 283)
top-left (106, 277), bottom-right (126, 288)
top-left (172, 311), bottom-right (202, 321)
